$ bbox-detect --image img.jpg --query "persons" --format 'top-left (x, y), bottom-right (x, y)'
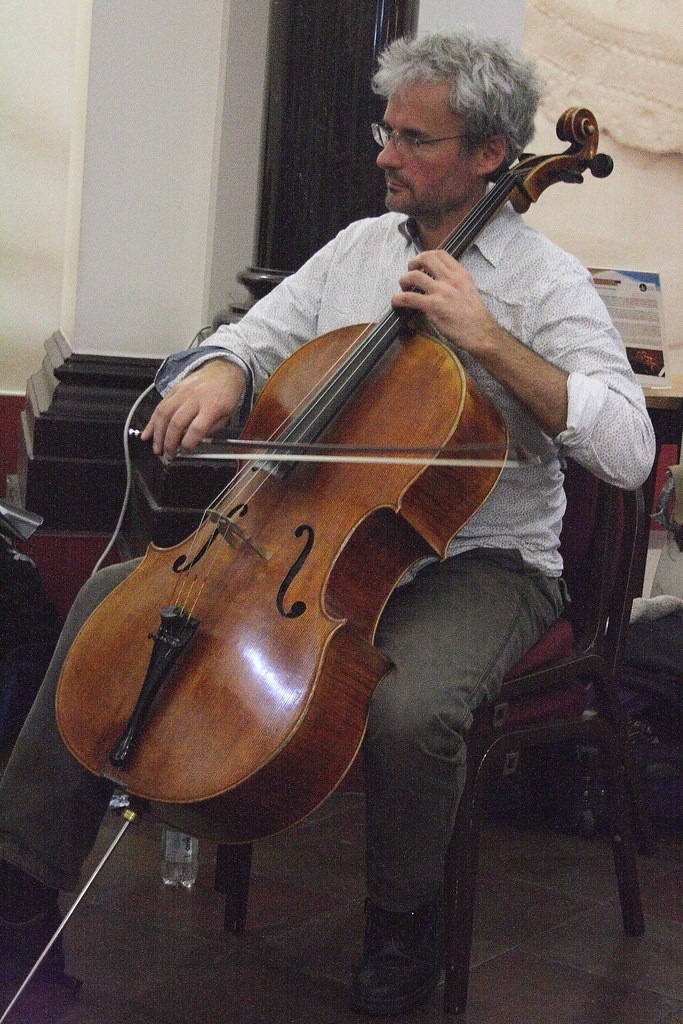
top-left (0, 25), bottom-right (656, 1024)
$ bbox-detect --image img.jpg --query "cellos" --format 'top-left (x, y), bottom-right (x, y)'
top-left (0, 106), bottom-right (616, 1023)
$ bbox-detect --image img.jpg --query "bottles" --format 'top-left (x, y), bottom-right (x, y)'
top-left (160, 826), bottom-right (198, 888)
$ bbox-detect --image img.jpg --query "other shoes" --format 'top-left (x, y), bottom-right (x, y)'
top-left (350, 890), bottom-right (442, 1016)
top-left (0, 901), bottom-right (65, 975)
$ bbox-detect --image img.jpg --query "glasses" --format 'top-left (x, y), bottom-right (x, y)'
top-left (371, 119), bottom-right (471, 157)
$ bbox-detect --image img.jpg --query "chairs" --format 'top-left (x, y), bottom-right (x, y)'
top-left (218, 456), bottom-right (648, 1015)
